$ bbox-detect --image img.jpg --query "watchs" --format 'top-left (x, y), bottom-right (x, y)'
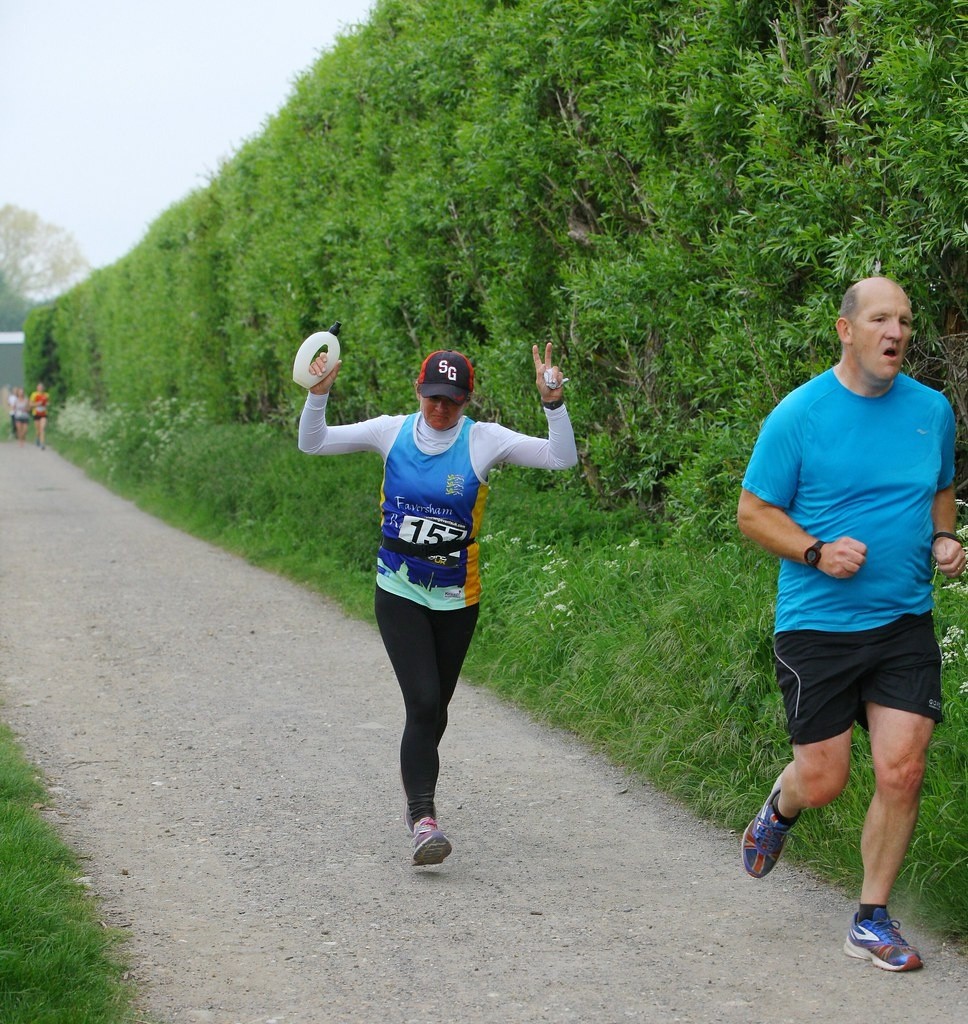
top-left (804, 540), bottom-right (826, 570)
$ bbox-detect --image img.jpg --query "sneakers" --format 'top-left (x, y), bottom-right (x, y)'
top-left (409, 817), bottom-right (452, 866)
top-left (842, 908), bottom-right (923, 971)
top-left (403, 793), bottom-right (414, 834)
top-left (741, 773), bottom-right (792, 879)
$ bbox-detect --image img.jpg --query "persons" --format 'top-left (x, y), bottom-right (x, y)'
top-left (738, 278), bottom-right (967, 971)
top-left (297, 343), bottom-right (578, 867)
top-left (1, 382), bottom-right (50, 451)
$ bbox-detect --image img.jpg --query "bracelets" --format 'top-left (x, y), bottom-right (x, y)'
top-left (541, 395), bottom-right (564, 411)
top-left (931, 531), bottom-right (964, 544)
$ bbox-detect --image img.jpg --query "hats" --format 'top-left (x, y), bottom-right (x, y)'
top-left (418, 349), bottom-right (475, 406)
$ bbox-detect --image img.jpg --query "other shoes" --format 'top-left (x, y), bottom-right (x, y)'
top-left (36, 437), bottom-right (45, 450)
